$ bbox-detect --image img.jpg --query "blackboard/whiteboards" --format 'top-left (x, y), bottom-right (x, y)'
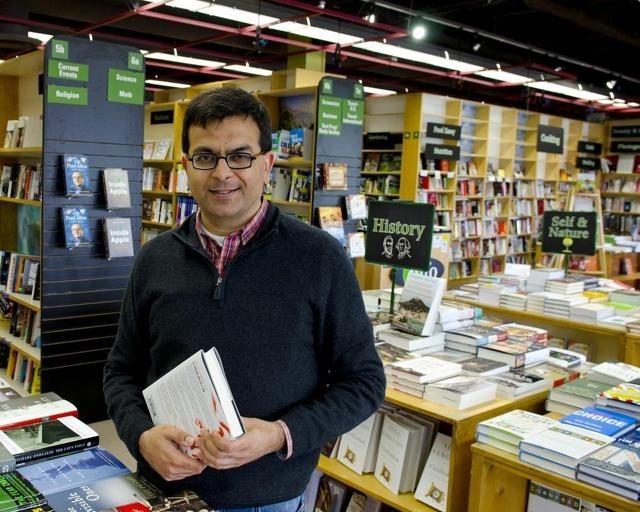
top-left (362, 200), bottom-right (436, 272)
top-left (540, 211), bottom-right (597, 256)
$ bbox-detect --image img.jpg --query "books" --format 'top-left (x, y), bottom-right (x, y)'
top-left (0, 162), bottom-right (43, 202)
top-left (416, 153), bottom-right (640, 279)
top-left (310, 266), bottom-right (640, 512)
top-left (59, 153), bottom-right (134, 258)
top-left (142, 348), bottom-right (246, 460)
top-left (262, 127), bottom-right (311, 223)
top-left (3, 117), bottom-right (30, 148)
top-left (318, 153), bottom-right (401, 258)
top-left (142, 137), bottom-right (198, 242)
top-left (0, 250), bottom-right (42, 395)
top-left (0, 376), bottom-right (211, 512)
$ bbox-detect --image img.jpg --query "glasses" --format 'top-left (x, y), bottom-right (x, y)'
top-left (187, 151), bottom-right (262, 170)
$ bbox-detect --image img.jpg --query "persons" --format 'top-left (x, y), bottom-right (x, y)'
top-left (98, 86), bottom-right (389, 511)
